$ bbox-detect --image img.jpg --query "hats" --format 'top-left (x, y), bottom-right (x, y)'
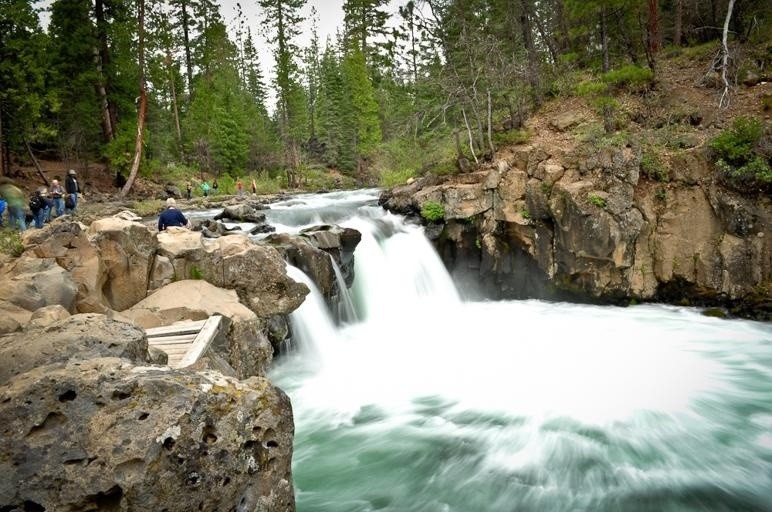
top-left (67, 170), bottom-right (77, 176)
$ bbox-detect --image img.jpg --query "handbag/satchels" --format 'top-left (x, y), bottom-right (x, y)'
top-left (65, 196), bottom-right (74, 208)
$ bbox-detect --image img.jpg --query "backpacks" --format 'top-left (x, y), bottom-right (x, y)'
top-left (30, 197), bottom-right (41, 211)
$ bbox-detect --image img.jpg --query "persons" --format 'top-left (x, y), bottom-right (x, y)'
top-left (155, 196), bottom-right (189, 233)
top-left (248, 179), bottom-right (256, 193)
top-left (210, 178), bottom-right (218, 197)
top-left (0, 169), bottom-right (81, 233)
top-left (236, 179), bottom-right (243, 195)
top-left (200, 180), bottom-right (209, 197)
top-left (185, 183), bottom-right (192, 201)
